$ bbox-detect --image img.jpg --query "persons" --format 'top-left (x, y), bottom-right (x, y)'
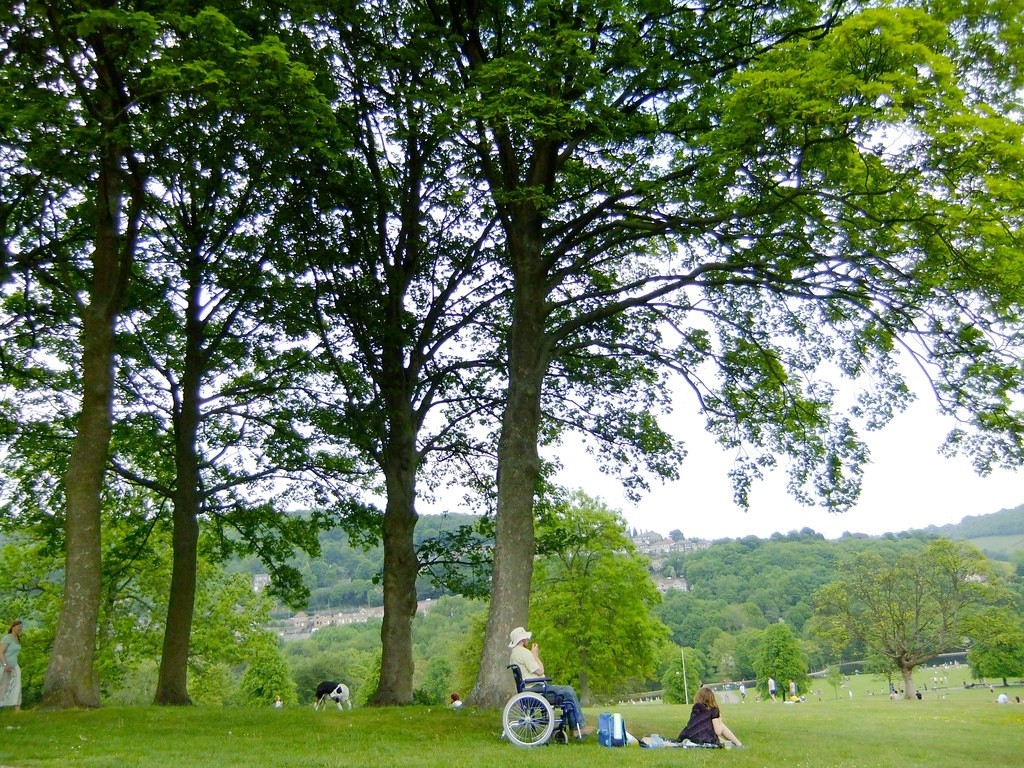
top-left (962, 678), bottom-right (966, 686)
top-left (767, 675), bottom-right (777, 704)
top-left (996, 692), bottom-right (1008, 704)
top-left (275, 696), bottom-right (283, 708)
top-left (507, 627), bottom-right (596, 739)
top-left (677, 688), bottom-right (744, 748)
top-left (789, 678), bottom-right (795, 697)
top-left (740, 683), bottom-right (746, 704)
top-left (450, 693), bottom-right (462, 708)
top-left (988, 684), bottom-right (994, 692)
top-left (0, 620), bottom-right (24, 713)
top-left (810, 660), bottom-right (960, 699)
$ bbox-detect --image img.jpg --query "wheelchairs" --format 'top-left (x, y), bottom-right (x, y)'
top-left (502, 663), bottom-right (587, 747)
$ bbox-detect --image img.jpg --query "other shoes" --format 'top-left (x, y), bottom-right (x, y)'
top-left (736, 744), bottom-right (748, 749)
top-left (570, 725), bottom-right (596, 737)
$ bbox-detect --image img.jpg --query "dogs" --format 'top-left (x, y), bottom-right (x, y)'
top-left (314, 681), bottom-right (351, 711)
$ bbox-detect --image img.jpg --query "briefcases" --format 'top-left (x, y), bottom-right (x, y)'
top-left (596, 713), bottom-right (629, 748)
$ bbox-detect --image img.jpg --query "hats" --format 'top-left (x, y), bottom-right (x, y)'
top-left (508, 627), bottom-right (532, 648)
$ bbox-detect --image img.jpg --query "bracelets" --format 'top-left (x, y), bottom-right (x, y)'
top-left (2, 663), bottom-right (8, 666)
